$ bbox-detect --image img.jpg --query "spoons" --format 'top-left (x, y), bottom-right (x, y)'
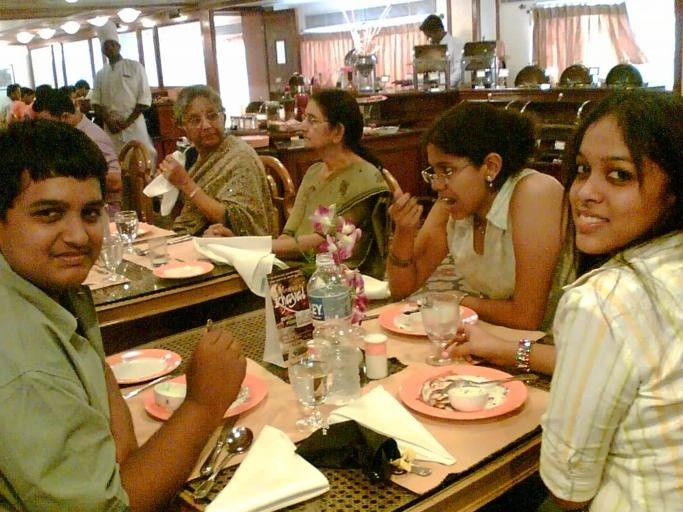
top-left (132, 236), bottom-right (194, 256)
top-left (444, 373), bottom-right (540, 396)
top-left (192, 426), bottom-right (253, 500)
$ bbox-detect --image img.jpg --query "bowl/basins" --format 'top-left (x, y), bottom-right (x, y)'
top-left (448, 387), bottom-right (489, 411)
top-left (153, 384), bottom-right (186, 411)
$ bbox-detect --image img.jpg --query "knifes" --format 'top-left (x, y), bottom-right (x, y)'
top-left (200, 413), bottom-right (238, 478)
top-left (122, 375), bottom-right (172, 399)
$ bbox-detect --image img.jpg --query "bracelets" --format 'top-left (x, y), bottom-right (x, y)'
top-left (386, 245), bottom-right (414, 268)
top-left (513, 337), bottom-right (539, 375)
top-left (187, 186), bottom-right (204, 200)
top-left (458, 292), bottom-right (472, 305)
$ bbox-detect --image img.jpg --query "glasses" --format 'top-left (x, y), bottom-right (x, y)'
top-left (182, 112), bottom-right (220, 123)
top-left (300, 113), bottom-right (328, 128)
top-left (421, 163), bottom-right (477, 183)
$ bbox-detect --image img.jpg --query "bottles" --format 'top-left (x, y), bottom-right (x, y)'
top-left (229, 115), bottom-right (256, 130)
top-left (283, 86), bottom-right (295, 123)
top-left (344, 71), bottom-right (356, 98)
top-left (307, 253), bottom-right (360, 405)
top-left (296, 83), bottom-right (308, 121)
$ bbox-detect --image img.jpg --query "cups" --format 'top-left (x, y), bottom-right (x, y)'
top-left (149, 236), bottom-right (168, 264)
top-left (357, 334), bottom-right (388, 380)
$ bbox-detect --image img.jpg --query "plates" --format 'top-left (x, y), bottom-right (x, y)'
top-left (152, 262), bottom-right (215, 279)
top-left (398, 365), bottom-right (529, 421)
top-left (379, 302), bottom-right (478, 335)
top-left (145, 372), bottom-right (269, 421)
top-left (106, 348), bottom-right (182, 383)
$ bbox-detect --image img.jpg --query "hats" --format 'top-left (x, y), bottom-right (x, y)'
top-left (96, 20), bottom-right (119, 44)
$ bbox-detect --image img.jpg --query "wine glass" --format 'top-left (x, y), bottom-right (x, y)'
top-left (115, 210), bottom-right (142, 255)
top-left (421, 294), bottom-right (458, 365)
top-left (288, 344), bottom-right (335, 433)
top-left (99, 236), bottom-right (124, 283)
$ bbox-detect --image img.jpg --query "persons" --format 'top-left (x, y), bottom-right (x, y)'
top-left (419, 14), bottom-right (468, 90)
top-left (381, 96), bottom-right (581, 332)
top-left (263, 85), bottom-right (394, 283)
top-left (436, 88), bottom-right (682, 511)
top-left (0, 116), bottom-right (249, 512)
top-left (146, 83), bottom-right (279, 247)
top-left (0, 14), bottom-right (162, 221)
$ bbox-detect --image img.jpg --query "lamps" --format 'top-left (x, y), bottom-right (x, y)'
top-left (13, 5), bottom-right (188, 45)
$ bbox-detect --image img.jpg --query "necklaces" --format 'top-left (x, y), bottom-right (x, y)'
top-left (473, 215), bottom-right (485, 237)
